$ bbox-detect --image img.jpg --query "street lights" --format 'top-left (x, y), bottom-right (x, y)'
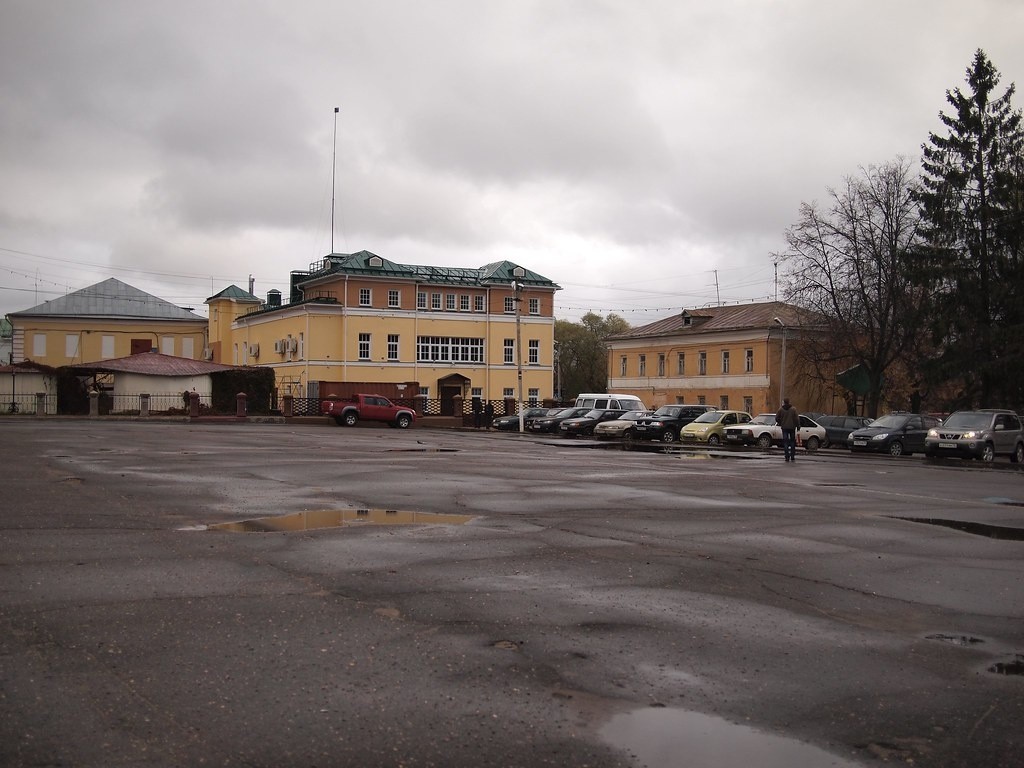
top-left (512, 280), bottom-right (526, 434)
top-left (775, 317), bottom-right (786, 409)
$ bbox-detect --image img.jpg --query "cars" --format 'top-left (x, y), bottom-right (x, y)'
top-left (680, 410), bottom-right (753, 446)
top-left (846, 414), bottom-right (943, 458)
top-left (631, 404), bottom-right (719, 444)
top-left (594, 410), bottom-right (657, 440)
top-left (559, 409), bottom-right (629, 437)
top-left (491, 408), bottom-right (593, 435)
top-left (722, 414), bottom-right (826, 453)
top-left (815, 414), bottom-right (876, 449)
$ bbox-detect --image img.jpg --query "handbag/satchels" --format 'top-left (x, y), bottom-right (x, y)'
top-left (797, 431), bottom-right (801, 445)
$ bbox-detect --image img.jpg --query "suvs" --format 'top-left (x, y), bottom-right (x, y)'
top-left (924, 409), bottom-right (1024, 463)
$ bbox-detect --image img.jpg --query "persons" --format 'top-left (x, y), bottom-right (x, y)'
top-left (649, 405), bottom-right (655, 411)
top-left (775, 398), bottom-right (800, 462)
top-left (673, 410), bottom-right (679, 415)
top-left (473, 401), bottom-right (484, 428)
top-left (484, 401), bottom-right (494, 429)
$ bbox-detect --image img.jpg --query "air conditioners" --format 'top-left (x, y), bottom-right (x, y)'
top-left (274, 340), bottom-right (285, 354)
top-left (249, 344), bottom-right (258, 357)
top-left (204, 349), bottom-right (212, 360)
top-left (286, 340), bottom-right (296, 352)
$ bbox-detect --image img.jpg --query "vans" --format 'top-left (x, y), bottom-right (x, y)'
top-left (574, 394), bottom-right (647, 412)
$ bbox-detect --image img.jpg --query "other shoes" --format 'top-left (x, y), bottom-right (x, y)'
top-left (785, 458), bottom-right (789, 461)
top-left (791, 456), bottom-right (795, 460)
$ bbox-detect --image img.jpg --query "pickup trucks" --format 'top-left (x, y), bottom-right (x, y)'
top-left (321, 394), bottom-right (416, 430)
